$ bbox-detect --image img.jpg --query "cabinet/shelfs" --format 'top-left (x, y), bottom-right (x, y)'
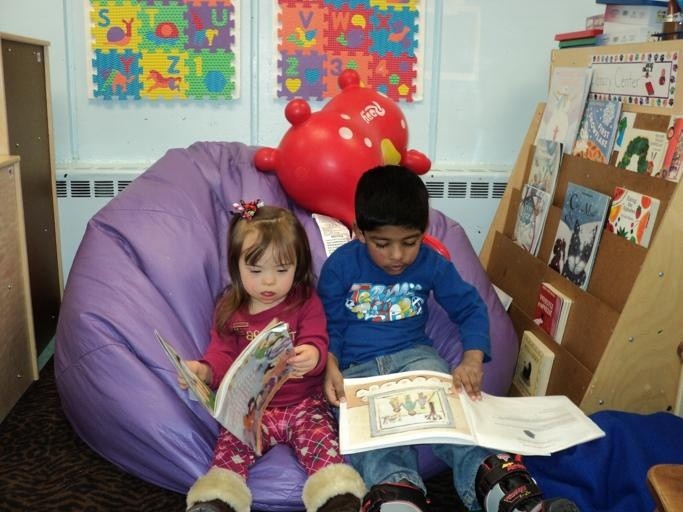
top-left (478, 38), bottom-right (680, 417)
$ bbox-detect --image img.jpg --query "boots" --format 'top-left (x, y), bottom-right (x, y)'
top-left (362, 482), bottom-right (428, 512)
top-left (474, 452), bottom-right (580, 512)
top-left (185, 466), bottom-right (253, 512)
top-left (301, 463), bottom-right (367, 512)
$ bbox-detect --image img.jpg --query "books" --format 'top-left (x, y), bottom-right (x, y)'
top-left (152, 317), bottom-right (304, 458)
top-left (336, 370), bottom-right (606, 457)
top-left (513, 67), bottom-right (682, 289)
